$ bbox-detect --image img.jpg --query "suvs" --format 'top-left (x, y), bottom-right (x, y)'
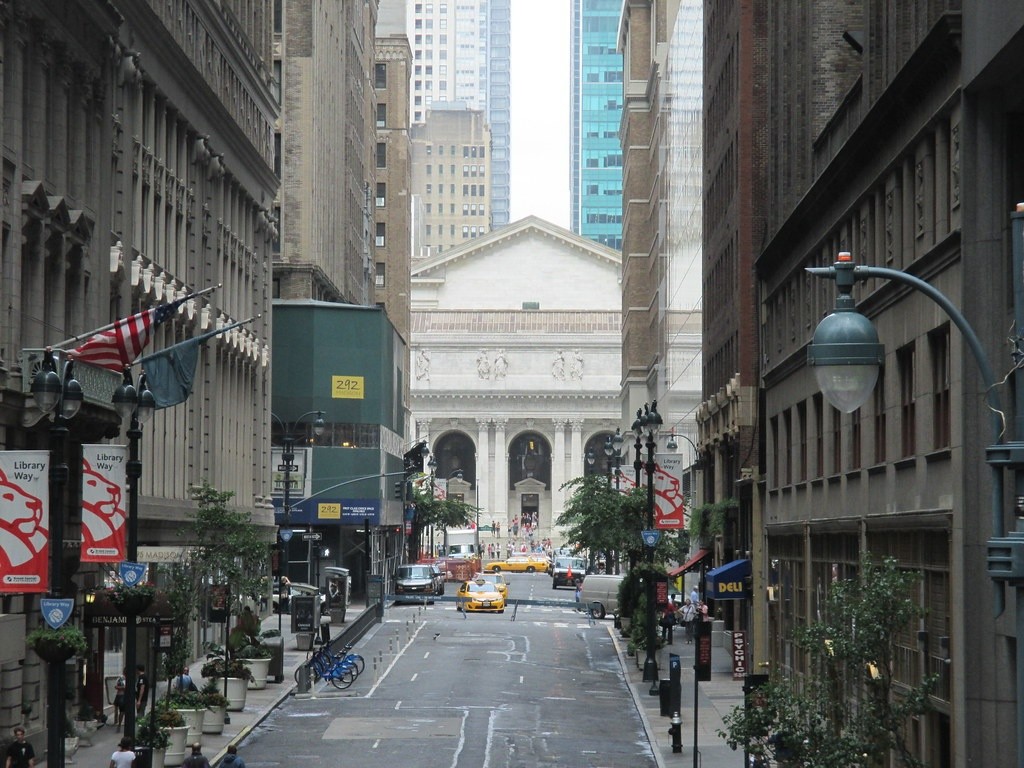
top-left (393, 565), bottom-right (438, 605)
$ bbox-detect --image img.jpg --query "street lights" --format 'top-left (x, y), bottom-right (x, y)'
top-left (603, 437), bottom-right (612, 575)
top-left (109, 367), bottom-right (156, 763)
top-left (806, 253), bottom-right (1023, 768)
top-left (613, 427), bottom-right (623, 574)
top-left (427, 456), bottom-right (438, 558)
top-left (632, 401), bottom-right (664, 682)
top-left (588, 448), bottom-right (595, 574)
top-left (21, 352), bottom-right (87, 768)
top-left (272, 409), bottom-right (327, 615)
top-left (444, 468), bottom-right (464, 555)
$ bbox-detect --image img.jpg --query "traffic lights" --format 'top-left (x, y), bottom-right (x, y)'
top-left (394, 480), bottom-right (405, 502)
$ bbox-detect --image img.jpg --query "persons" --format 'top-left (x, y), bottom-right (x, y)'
top-left (662, 585), bottom-right (708, 645)
top-left (173, 667), bottom-right (192, 693)
top-left (437, 542), bottom-right (441, 549)
top-left (6, 727), bottom-right (35, 768)
top-left (181, 742), bottom-right (212, 768)
top-left (219, 745), bottom-right (246, 768)
top-left (110, 736), bottom-right (136, 768)
top-left (465, 514), bottom-right (475, 529)
top-left (477, 512), bottom-right (552, 559)
top-left (112, 664), bottom-right (149, 734)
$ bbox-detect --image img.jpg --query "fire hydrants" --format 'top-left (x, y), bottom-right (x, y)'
top-left (668, 714), bottom-right (684, 753)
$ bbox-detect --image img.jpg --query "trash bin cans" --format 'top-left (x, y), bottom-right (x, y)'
top-left (660, 679), bottom-right (681, 716)
top-left (259, 636), bottom-right (284, 683)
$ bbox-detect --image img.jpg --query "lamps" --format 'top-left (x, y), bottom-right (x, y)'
top-left (842, 30), bottom-right (864, 54)
top-left (83, 589), bottom-right (95, 608)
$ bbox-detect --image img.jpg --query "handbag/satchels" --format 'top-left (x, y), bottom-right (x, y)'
top-left (681, 620), bottom-right (686, 627)
top-left (659, 617), bottom-right (669, 627)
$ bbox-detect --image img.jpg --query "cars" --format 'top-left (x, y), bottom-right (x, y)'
top-left (272, 582), bottom-right (326, 613)
top-left (434, 543), bottom-right (549, 573)
top-left (433, 565), bottom-right (445, 595)
top-left (456, 572), bottom-right (511, 615)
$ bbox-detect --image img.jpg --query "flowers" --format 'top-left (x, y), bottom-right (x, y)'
top-left (106, 582), bottom-right (156, 603)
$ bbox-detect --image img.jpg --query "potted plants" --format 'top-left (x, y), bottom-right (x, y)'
top-left (235, 606), bottom-right (274, 689)
top-left (200, 640), bottom-right (259, 711)
top-left (71, 702), bottom-right (98, 747)
top-left (196, 677), bottom-right (232, 734)
top-left (616, 572), bottom-right (641, 631)
top-left (155, 687), bottom-right (208, 747)
top-left (135, 710), bottom-right (173, 768)
top-left (65, 713), bottom-right (80, 764)
top-left (636, 636), bottom-right (665, 671)
top-left (137, 702), bottom-right (191, 766)
top-left (23, 621), bottom-right (89, 664)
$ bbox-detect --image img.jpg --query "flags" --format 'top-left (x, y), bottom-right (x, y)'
top-left (73, 301), bottom-right (180, 374)
top-left (142, 339), bottom-right (200, 410)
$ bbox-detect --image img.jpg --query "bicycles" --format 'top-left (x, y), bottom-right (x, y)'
top-left (294, 642), bottom-right (367, 688)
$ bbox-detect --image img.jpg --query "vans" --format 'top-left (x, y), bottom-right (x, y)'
top-left (545, 547), bottom-right (587, 590)
top-left (578, 573), bottom-right (627, 619)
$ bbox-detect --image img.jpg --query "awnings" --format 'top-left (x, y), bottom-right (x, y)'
top-left (705, 558), bottom-right (753, 601)
top-left (665, 548), bottom-right (710, 580)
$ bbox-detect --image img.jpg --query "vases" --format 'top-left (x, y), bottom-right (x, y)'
top-left (109, 595), bottom-right (154, 615)
